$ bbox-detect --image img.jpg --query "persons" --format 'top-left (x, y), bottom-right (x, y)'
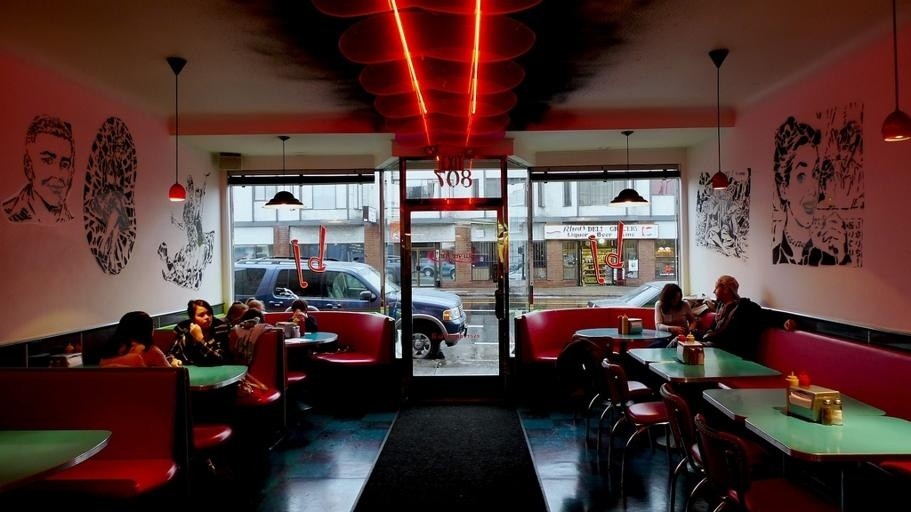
top-left (239, 307), bottom-right (265, 325)
top-left (164, 300), bottom-right (225, 368)
top-left (666, 276), bottom-right (796, 354)
top-left (770, 116), bottom-right (853, 264)
top-left (224, 302), bottom-right (248, 325)
top-left (648, 284), bottom-right (696, 348)
top-left (287, 300), bottom-right (318, 333)
top-left (2, 114), bottom-right (76, 225)
top-left (99, 311), bottom-right (174, 368)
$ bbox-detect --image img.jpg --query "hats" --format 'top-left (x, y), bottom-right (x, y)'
top-left (716, 275), bottom-right (741, 298)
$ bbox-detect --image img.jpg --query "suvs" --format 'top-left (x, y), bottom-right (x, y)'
top-left (386, 255), bottom-right (400, 285)
top-left (235, 259), bottom-right (468, 359)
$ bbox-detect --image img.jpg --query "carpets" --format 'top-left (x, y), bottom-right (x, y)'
top-left (348, 401), bottom-right (552, 511)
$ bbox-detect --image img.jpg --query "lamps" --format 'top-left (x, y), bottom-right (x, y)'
top-left (881, 0), bottom-right (911, 142)
top-left (263, 135), bottom-right (304, 211)
top-left (610, 131), bottom-right (648, 207)
top-left (708, 48), bottom-right (732, 191)
top-left (164, 54), bottom-right (192, 202)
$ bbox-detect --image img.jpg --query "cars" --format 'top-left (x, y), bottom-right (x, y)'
top-left (416, 257), bottom-right (455, 279)
top-left (587, 281), bottom-right (678, 307)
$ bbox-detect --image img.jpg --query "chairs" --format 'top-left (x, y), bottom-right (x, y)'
top-left (687, 411), bottom-right (819, 512)
top-left (598, 357), bottom-right (670, 507)
top-left (661, 380), bottom-right (767, 509)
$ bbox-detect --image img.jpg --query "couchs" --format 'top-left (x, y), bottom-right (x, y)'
top-left (0, 366), bottom-right (183, 498)
top-left (150, 333), bottom-right (284, 406)
top-left (514, 292), bottom-right (911, 490)
top-left (184, 369), bottom-right (234, 451)
top-left (262, 310), bottom-right (396, 368)
top-left (283, 330), bottom-right (307, 385)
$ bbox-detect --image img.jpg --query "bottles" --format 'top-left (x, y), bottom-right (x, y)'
top-left (799, 372), bottom-right (809, 386)
top-left (621, 314), bottom-right (628, 334)
top-left (687, 331), bottom-right (695, 341)
top-left (784, 371), bottom-right (799, 413)
top-left (677, 331), bottom-right (685, 341)
top-left (617, 313), bottom-right (622, 334)
top-left (66, 343), bottom-right (73, 352)
